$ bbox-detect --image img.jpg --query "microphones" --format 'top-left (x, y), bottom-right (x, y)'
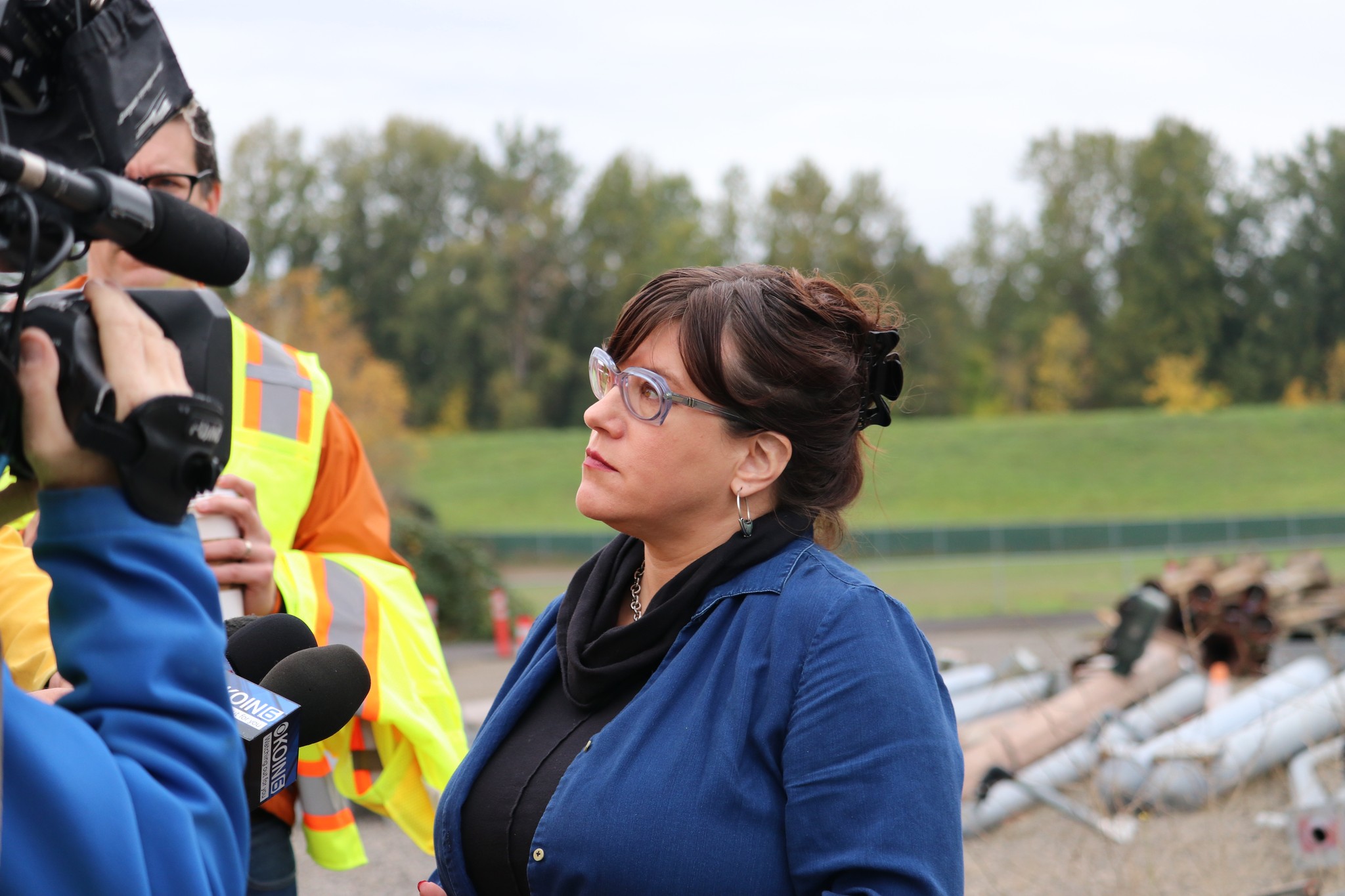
top-left (227, 608), bottom-right (322, 691)
top-left (0, 140), bottom-right (253, 290)
top-left (225, 640), bottom-right (371, 819)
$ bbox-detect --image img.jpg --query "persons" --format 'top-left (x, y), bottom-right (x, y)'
top-left (1, 279), bottom-right (250, 894)
top-left (416, 262), bottom-right (965, 895)
top-left (1, 92), bottom-right (469, 896)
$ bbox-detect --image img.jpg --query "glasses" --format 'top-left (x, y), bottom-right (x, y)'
top-left (128, 167), bottom-right (215, 206)
top-left (587, 346), bottom-right (760, 432)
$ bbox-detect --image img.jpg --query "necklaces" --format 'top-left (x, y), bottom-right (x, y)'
top-left (631, 561), bottom-right (645, 623)
